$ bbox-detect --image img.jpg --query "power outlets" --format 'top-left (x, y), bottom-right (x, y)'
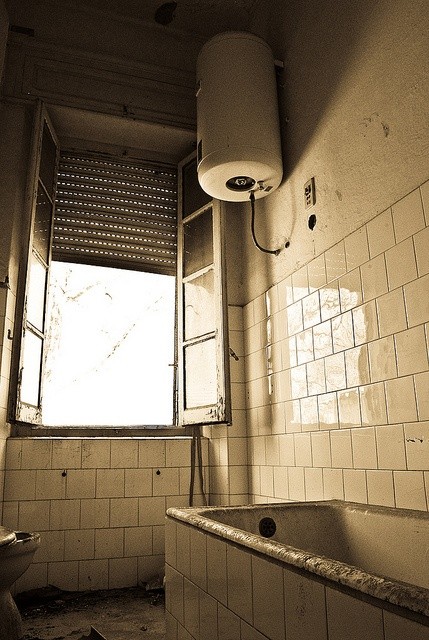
top-left (301, 178), bottom-right (315, 212)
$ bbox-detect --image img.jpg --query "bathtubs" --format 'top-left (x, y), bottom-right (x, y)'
top-left (164, 500), bottom-right (429, 640)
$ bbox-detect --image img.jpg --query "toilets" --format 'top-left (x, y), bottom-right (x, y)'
top-left (0, 529), bottom-right (40, 638)
